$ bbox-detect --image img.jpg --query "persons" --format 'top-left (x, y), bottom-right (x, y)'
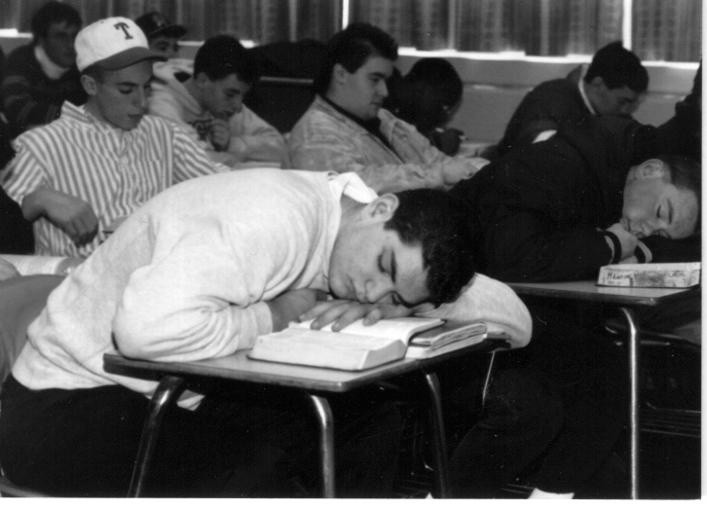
top-left (0, 1), bottom-right (88, 166)
top-left (439, 132), bottom-right (700, 498)
top-left (0, 166), bottom-right (535, 498)
top-left (143, 33), bottom-right (286, 172)
top-left (0, 14), bottom-right (234, 262)
top-left (134, 11), bottom-right (187, 59)
top-left (475, 40), bottom-right (649, 164)
top-left (287, 20), bottom-right (490, 194)
top-left (380, 56), bottom-right (465, 158)
top-left (658, 61), bottom-right (701, 164)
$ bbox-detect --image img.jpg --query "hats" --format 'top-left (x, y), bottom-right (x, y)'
top-left (73, 15), bottom-right (169, 76)
top-left (134, 11), bottom-right (188, 41)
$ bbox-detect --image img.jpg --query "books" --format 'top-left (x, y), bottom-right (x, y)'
top-left (594, 261), bottom-right (701, 289)
top-left (246, 316), bottom-right (488, 372)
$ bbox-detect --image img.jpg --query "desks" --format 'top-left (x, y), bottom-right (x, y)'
top-left (495, 256), bottom-right (700, 501)
top-left (94, 315), bottom-right (518, 498)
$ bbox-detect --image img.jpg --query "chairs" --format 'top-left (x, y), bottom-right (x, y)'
top-left (1, 272), bottom-right (75, 386)
